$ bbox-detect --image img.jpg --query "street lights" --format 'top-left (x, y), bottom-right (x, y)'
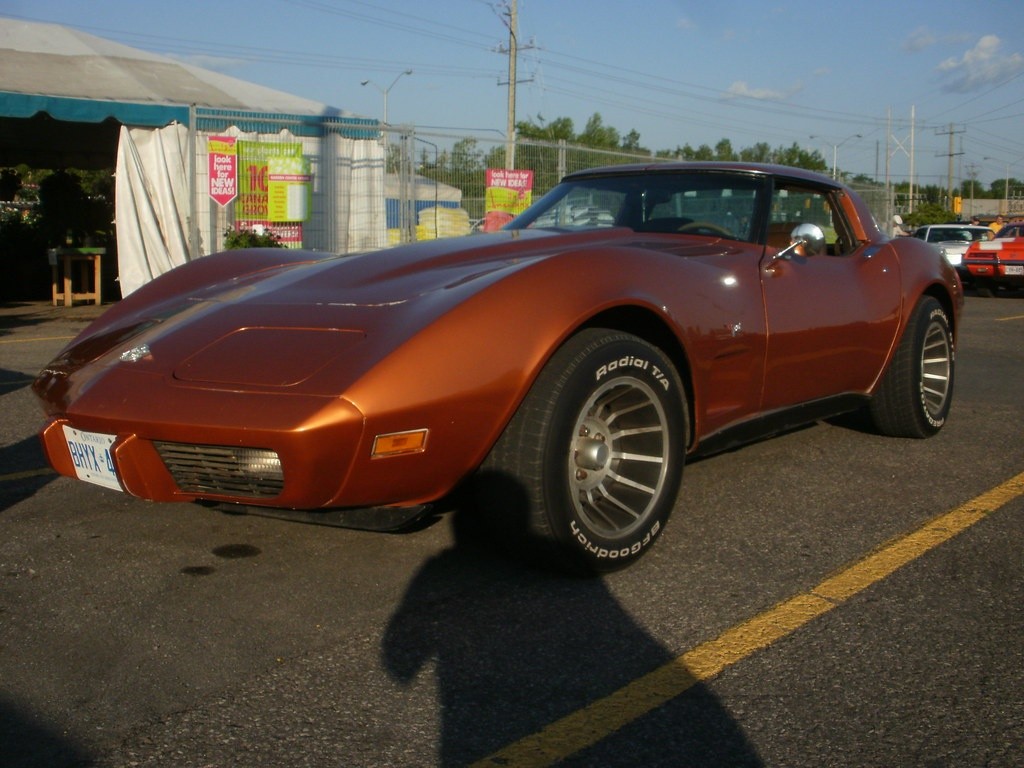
top-left (983, 156), bottom-right (1024, 215)
top-left (358, 69), bottom-right (414, 178)
top-left (807, 133), bottom-right (864, 227)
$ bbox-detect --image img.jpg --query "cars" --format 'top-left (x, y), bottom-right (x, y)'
top-left (962, 221), bottom-right (1024, 292)
top-left (912, 222), bottom-right (998, 280)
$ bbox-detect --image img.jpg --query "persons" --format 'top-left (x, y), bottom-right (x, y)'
top-left (969, 215), bottom-right (980, 226)
top-left (986, 215), bottom-right (1005, 241)
top-left (883, 215), bottom-right (910, 239)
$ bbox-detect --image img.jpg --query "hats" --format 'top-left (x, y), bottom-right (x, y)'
top-left (893, 215), bottom-right (903, 224)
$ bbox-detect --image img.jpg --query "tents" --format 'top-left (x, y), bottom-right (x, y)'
top-left (0, 17), bottom-right (462, 300)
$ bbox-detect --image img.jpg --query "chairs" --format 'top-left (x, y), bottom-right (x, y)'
top-left (763, 221), bottom-right (804, 248)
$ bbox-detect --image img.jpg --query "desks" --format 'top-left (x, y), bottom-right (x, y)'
top-left (47, 245), bottom-right (106, 307)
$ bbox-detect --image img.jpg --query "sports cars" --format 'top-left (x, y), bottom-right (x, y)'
top-left (29, 159), bottom-right (968, 583)
